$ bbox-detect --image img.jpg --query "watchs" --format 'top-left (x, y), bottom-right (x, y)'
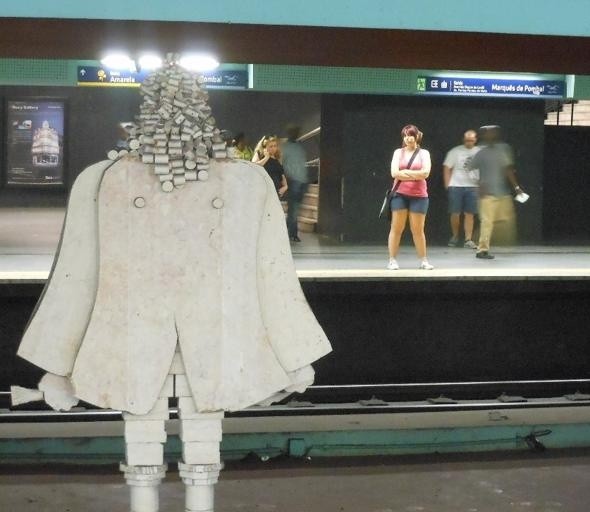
top-left (514, 185), bottom-right (522, 191)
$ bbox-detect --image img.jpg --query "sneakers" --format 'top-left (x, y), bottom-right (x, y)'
top-left (463, 240), bottom-right (478, 249)
top-left (387, 258), bottom-right (400, 270)
top-left (447, 235), bottom-right (459, 248)
top-left (419, 259), bottom-right (434, 270)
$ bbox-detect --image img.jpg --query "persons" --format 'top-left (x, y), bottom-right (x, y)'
top-left (468, 125), bottom-right (526, 259)
top-left (251, 134), bottom-right (288, 201)
top-left (443, 129), bottom-right (481, 249)
top-left (388, 124), bottom-right (433, 269)
top-left (232, 133), bottom-right (255, 161)
top-left (282, 128), bottom-right (308, 241)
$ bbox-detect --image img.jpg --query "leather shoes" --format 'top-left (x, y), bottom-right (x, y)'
top-left (476, 252), bottom-right (496, 259)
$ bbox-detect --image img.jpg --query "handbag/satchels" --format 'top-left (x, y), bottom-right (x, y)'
top-left (378, 188), bottom-right (394, 220)
